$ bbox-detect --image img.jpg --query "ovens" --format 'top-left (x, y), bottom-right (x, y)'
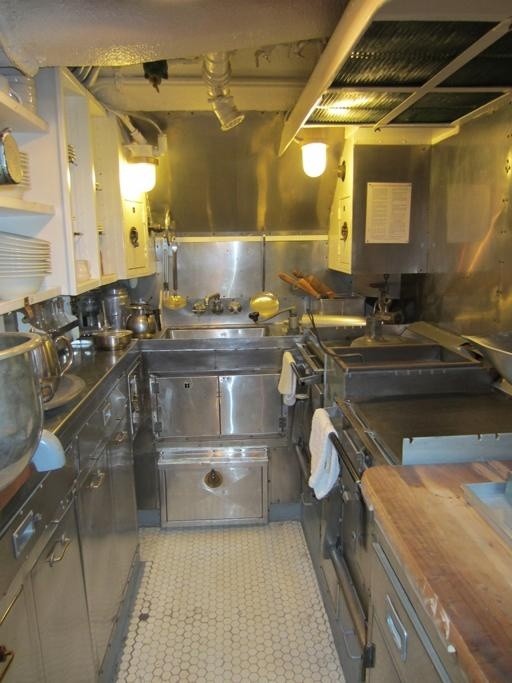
top-left (286, 340), bottom-right (370, 682)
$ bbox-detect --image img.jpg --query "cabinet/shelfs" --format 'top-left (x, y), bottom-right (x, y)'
top-left (69, 375), bottom-right (141, 655)
top-left (0, 447), bottom-right (101, 683)
top-left (326, 146), bottom-right (435, 275)
top-left (56, 65), bottom-right (120, 297)
top-left (360, 509), bottom-right (470, 681)
top-left (0, 39), bottom-right (68, 314)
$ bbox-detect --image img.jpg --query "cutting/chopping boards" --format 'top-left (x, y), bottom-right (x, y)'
top-left (360, 458), bottom-right (511, 682)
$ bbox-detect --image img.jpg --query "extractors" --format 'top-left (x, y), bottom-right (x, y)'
top-left (301, 14), bottom-right (511, 126)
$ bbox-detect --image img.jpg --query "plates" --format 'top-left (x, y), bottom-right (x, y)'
top-left (1, 128), bottom-right (33, 199)
top-left (41, 372), bottom-right (85, 411)
top-left (98, 220), bottom-right (105, 232)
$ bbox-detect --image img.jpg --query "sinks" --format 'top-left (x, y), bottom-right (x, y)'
top-left (159, 323), bottom-right (271, 340)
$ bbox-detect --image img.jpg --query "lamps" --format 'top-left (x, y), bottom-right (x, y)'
top-left (122, 140), bottom-right (159, 193)
top-left (300, 137), bottom-right (346, 182)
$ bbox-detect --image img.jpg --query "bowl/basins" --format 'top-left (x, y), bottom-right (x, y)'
top-left (91, 329), bottom-right (131, 349)
top-left (0, 228), bottom-right (51, 300)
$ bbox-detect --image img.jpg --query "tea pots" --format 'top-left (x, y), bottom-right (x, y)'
top-left (126, 301), bottom-right (158, 338)
top-left (29, 328), bottom-right (75, 404)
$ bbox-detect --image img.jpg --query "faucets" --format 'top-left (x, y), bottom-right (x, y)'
top-left (203, 292), bottom-right (224, 315)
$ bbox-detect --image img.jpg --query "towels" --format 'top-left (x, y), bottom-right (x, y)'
top-left (278, 349), bottom-right (300, 407)
top-left (306, 406), bottom-right (341, 500)
